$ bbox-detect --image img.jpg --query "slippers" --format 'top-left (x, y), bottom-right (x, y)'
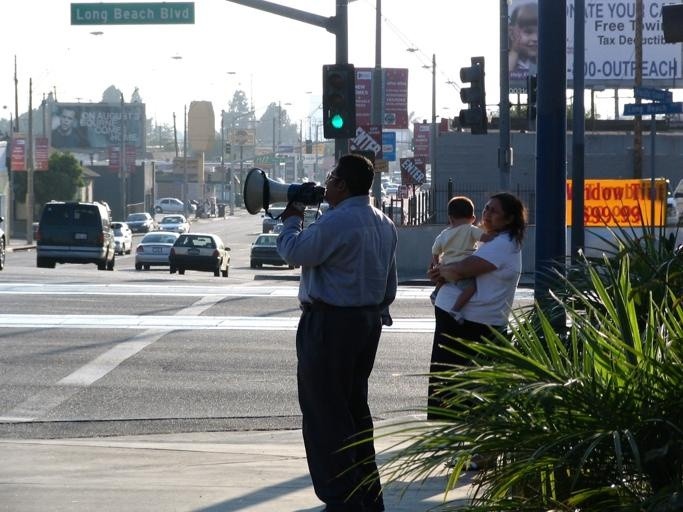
top-left (449, 458), bottom-right (497, 470)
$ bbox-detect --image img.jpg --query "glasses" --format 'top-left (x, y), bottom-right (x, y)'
top-left (326, 172), bottom-right (343, 184)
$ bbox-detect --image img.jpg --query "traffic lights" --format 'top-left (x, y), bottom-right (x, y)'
top-left (526, 70), bottom-right (536, 122)
top-left (321, 63), bottom-right (357, 140)
top-left (459, 54), bottom-right (489, 137)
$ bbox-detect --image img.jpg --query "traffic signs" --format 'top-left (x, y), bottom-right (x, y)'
top-left (621, 100), bottom-right (683, 116)
top-left (66, 1), bottom-right (196, 26)
top-left (632, 86), bottom-right (674, 103)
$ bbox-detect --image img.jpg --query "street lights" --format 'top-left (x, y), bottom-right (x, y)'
top-left (218, 66), bottom-right (258, 158)
top-left (2, 104), bottom-right (14, 134)
top-left (420, 62), bottom-right (438, 214)
top-left (444, 79), bottom-right (459, 88)
top-left (277, 101), bottom-right (292, 145)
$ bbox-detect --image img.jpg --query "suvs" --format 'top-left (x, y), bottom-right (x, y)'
top-left (32, 195), bottom-right (116, 273)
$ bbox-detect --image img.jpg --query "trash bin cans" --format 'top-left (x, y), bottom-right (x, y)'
top-left (217, 203), bottom-right (226, 217)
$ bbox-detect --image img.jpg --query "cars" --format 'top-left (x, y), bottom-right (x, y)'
top-left (246, 165), bottom-right (431, 273)
top-left (489, 110), bottom-right (499, 129)
top-left (0, 215), bottom-right (7, 273)
top-left (639, 176), bottom-right (680, 227)
top-left (105, 194), bottom-right (231, 280)
top-left (671, 178), bottom-right (682, 223)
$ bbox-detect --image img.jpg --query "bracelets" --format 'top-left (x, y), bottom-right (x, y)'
top-left (435, 263), bottom-right (441, 272)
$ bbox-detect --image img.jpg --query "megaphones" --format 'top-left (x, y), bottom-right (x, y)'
top-left (243, 167), bottom-right (324, 215)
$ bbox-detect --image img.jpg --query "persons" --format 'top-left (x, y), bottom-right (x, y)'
top-left (427, 191), bottom-right (530, 471)
top-left (429, 197), bottom-right (499, 327)
top-left (507, 2), bottom-right (539, 77)
top-left (277, 156), bottom-right (399, 512)
